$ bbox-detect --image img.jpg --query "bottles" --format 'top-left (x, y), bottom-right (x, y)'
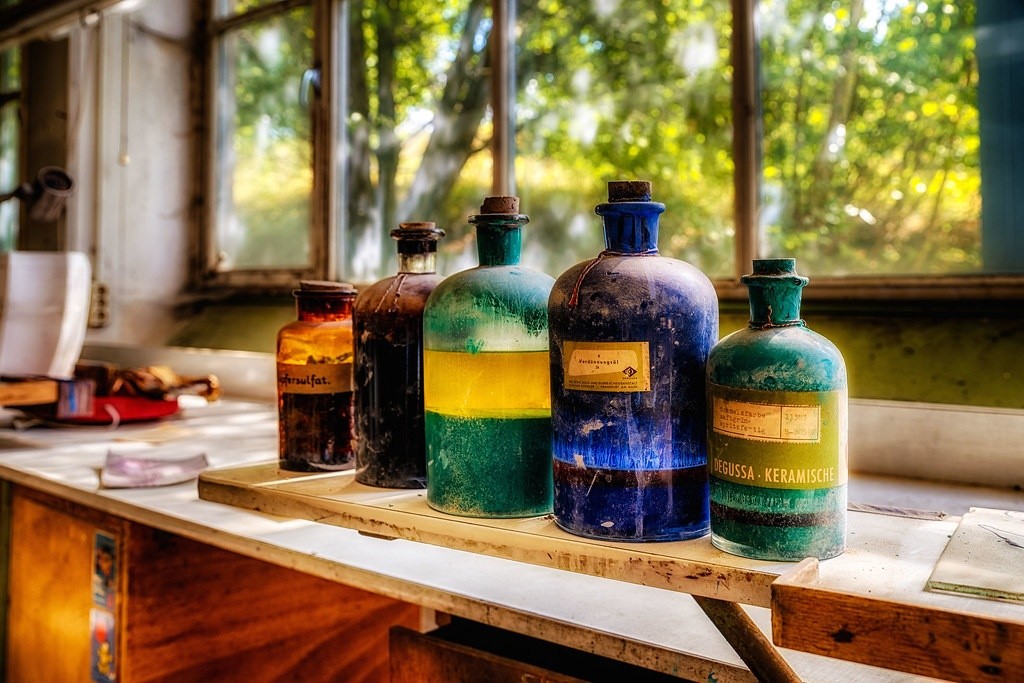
top-left (275, 280), bottom-right (358, 473)
top-left (704, 257), bottom-right (847, 562)
top-left (351, 220), bottom-right (446, 488)
top-left (423, 196), bottom-right (558, 519)
top-left (547, 181), bottom-right (720, 545)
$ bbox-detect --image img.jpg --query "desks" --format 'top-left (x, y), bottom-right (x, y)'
top-left (0, 400), bottom-right (1024, 683)
